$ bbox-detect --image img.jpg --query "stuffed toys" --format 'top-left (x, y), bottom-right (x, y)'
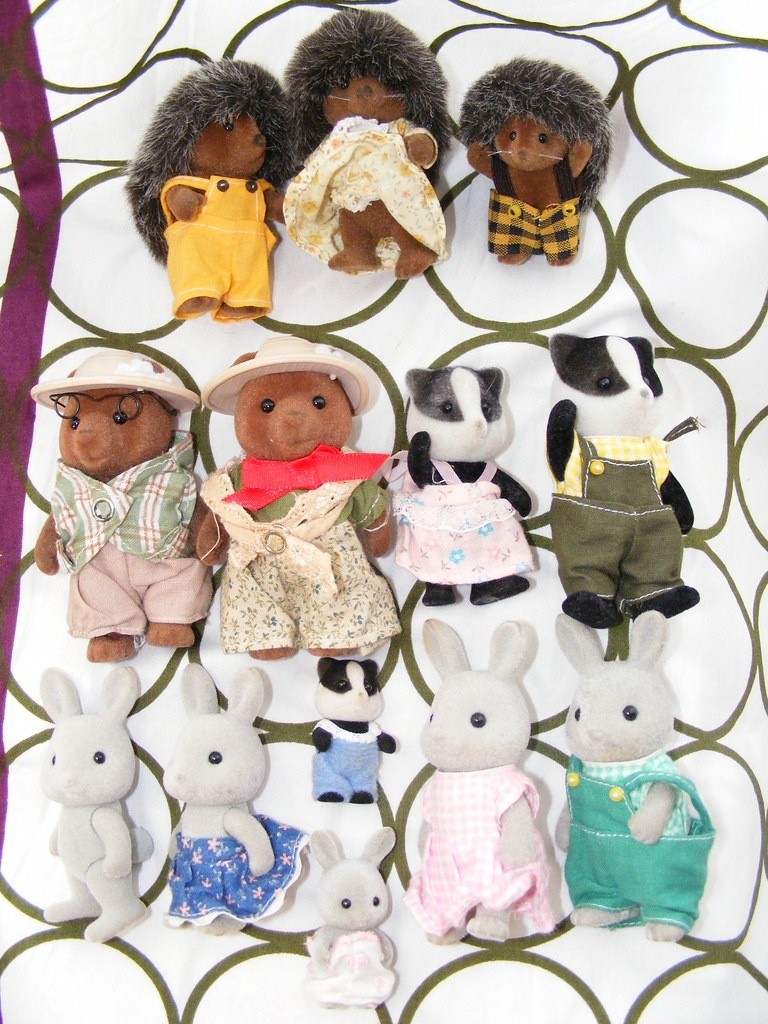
top-left (196, 337), bottom-right (401, 661)
top-left (459, 58), bottom-right (612, 265)
top-left (307, 826), bottom-right (397, 1011)
top-left (125, 62), bottom-right (291, 321)
top-left (41, 667), bottom-right (154, 941)
top-left (311, 656), bottom-right (396, 804)
top-left (282, 9), bottom-right (450, 279)
top-left (162, 663), bottom-right (310, 935)
top-left (555, 610), bottom-right (716, 942)
top-left (404, 619), bottom-right (555, 944)
top-left (394, 367), bottom-right (535, 606)
top-left (545, 332), bottom-right (701, 630)
top-left (31, 354), bottom-right (226, 663)
